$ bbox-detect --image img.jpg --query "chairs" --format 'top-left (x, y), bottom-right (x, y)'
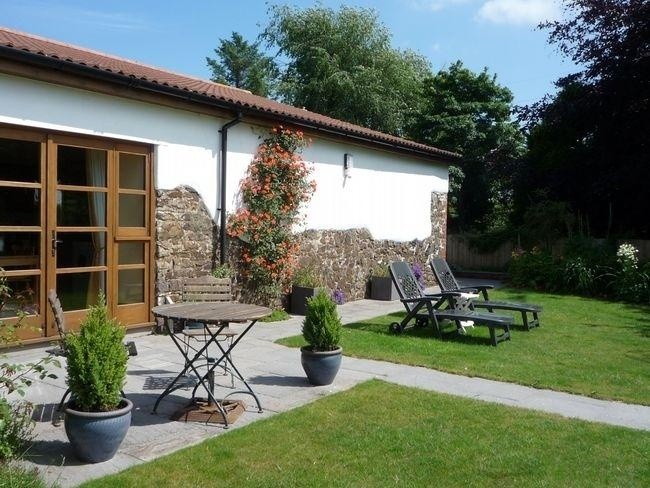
top-left (47, 287), bottom-right (137, 411)
top-left (388, 261), bottom-right (514, 347)
top-left (429, 258), bottom-right (542, 331)
top-left (182, 275), bottom-right (238, 390)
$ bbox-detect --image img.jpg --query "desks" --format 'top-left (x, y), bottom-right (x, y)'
top-left (150, 303), bottom-right (273, 429)
top-left (451, 291), bottom-right (480, 333)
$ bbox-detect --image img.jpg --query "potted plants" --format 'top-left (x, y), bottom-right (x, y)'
top-left (64, 289), bottom-right (133, 463)
top-left (300, 286), bottom-right (343, 385)
